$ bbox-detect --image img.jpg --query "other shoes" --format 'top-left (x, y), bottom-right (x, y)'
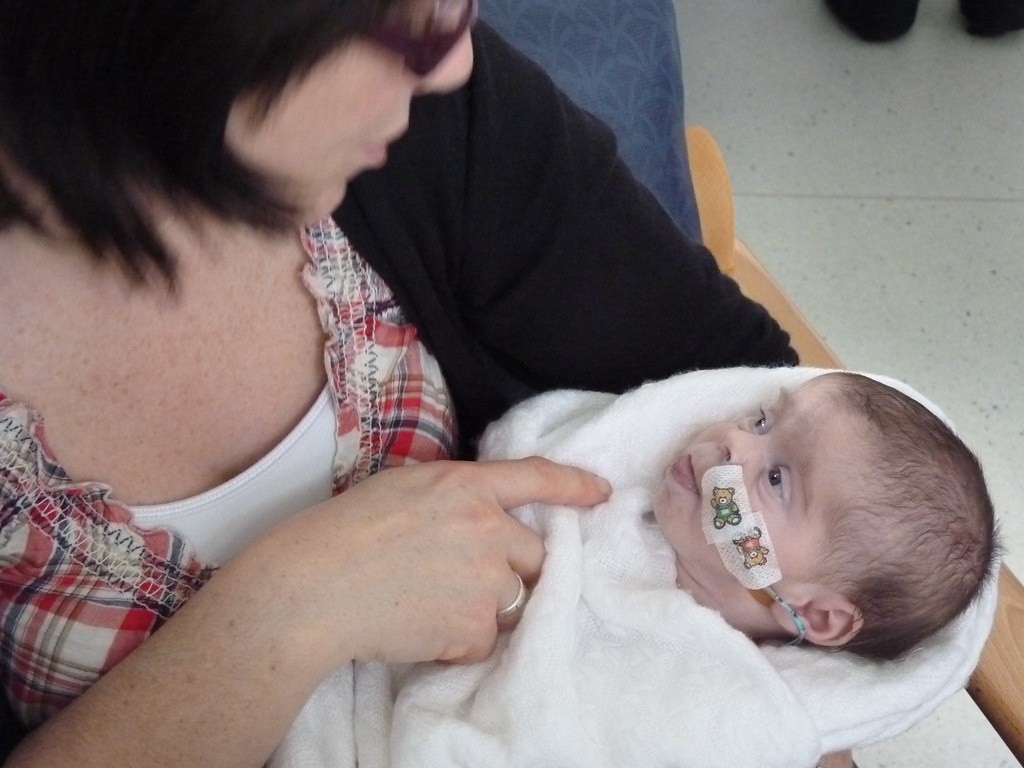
top-left (819, 2), bottom-right (1023, 44)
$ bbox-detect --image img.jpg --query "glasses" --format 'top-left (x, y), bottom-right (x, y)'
top-left (360, 0), bottom-right (476, 76)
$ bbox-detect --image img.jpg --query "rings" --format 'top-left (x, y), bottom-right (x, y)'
top-left (496, 570), bottom-right (527, 620)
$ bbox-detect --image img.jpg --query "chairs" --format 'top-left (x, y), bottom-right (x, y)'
top-left (478, 0), bottom-right (1024, 768)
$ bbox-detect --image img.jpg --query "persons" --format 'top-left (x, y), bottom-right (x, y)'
top-left (1, 0), bottom-right (858, 768)
top-left (822, 1), bottom-right (1024, 44)
top-left (267, 366), bottom-right (1004, 768)
